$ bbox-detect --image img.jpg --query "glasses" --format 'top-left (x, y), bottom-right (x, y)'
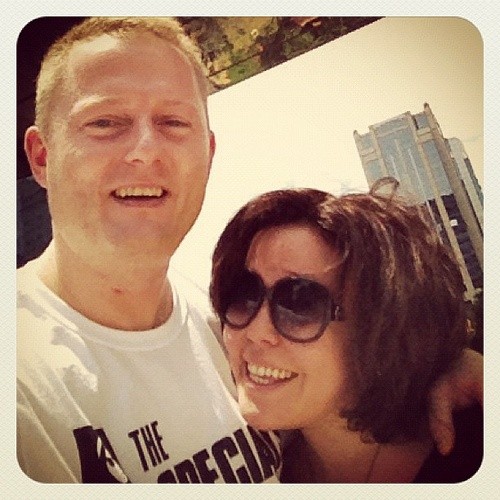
top-left (211, 263), bottom-right (348, 343)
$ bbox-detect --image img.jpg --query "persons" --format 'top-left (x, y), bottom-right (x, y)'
top-left (207, 187), bottom-right (485, 483)
top-left (13, 15), bottom-right (483, 486)
